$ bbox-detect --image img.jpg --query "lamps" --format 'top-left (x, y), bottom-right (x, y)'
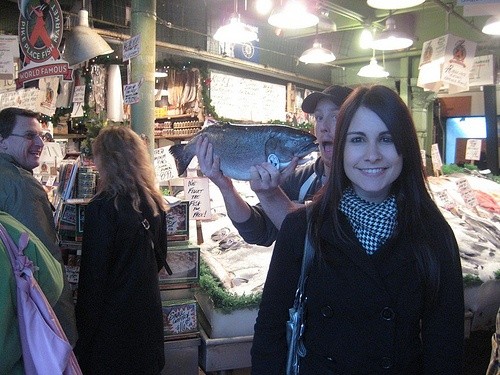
top-left (213, 0), bottom-right (258, 43)
top-left (371, 9), bottom-right (414, 50)
top-left (64, 0), bottom-right (115, 67)
top-left (482, 15), bottom-right (500, 36)
top-left (357, 49), bottom-right (390, 78)
top-left (299, 24), bottom-right (336, 63)
top-left (318, 10), bottom-right (337, 32)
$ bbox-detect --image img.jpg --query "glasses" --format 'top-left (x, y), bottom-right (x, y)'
top-left (9, 132), bottom-right (47, 142)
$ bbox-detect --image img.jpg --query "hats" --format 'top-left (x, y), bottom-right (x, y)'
top-left (301, 84), bottom-right (354, 113)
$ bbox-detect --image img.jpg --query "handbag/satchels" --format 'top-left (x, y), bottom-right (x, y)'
top-left (0, 223), bottom-right (82, 375)
top-left (284, 199), bottom-right (316, 375)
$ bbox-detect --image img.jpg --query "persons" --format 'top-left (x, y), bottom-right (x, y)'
top-left (0, 108), bottom-right (80, 350)
top-left (74, 125), bottom-right (168, 375)
top-left (250, 85), bottom-right (467, 375)
top-left (0, 210), bottom-right (65, 375)
top-left (195, 83), bottom-right (354, 247)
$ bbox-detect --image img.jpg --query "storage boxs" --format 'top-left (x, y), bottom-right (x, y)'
top-left (156, 241), bottom-right (200, 285)
top-left (161, 298), bottom-right (199, 335)
top-left (165, 201), bottom-right (189, 234)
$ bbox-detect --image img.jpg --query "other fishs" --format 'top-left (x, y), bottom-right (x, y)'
top-left (428, 176), bottom-right (500, 283)
top-left (168, 122), bottom-right (319, 181)
top-left (178, 184), bottom-right (268, 296)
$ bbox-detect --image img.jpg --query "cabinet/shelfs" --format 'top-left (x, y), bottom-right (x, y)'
top-left (60, 198), bottom-right (200, 375)
